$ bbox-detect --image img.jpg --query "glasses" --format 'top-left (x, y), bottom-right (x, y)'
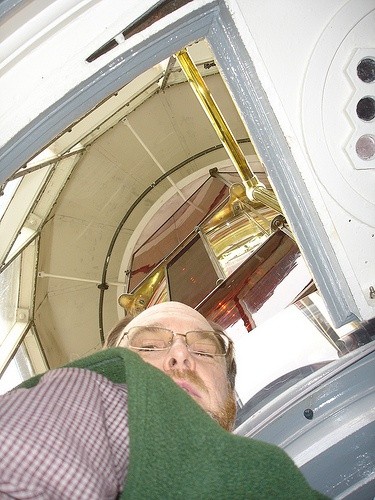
top-left (117, 326), bottom-right (228, 366)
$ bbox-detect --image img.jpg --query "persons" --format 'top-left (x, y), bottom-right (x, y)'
top-left (1, 300), bottom-right (335, 499)
